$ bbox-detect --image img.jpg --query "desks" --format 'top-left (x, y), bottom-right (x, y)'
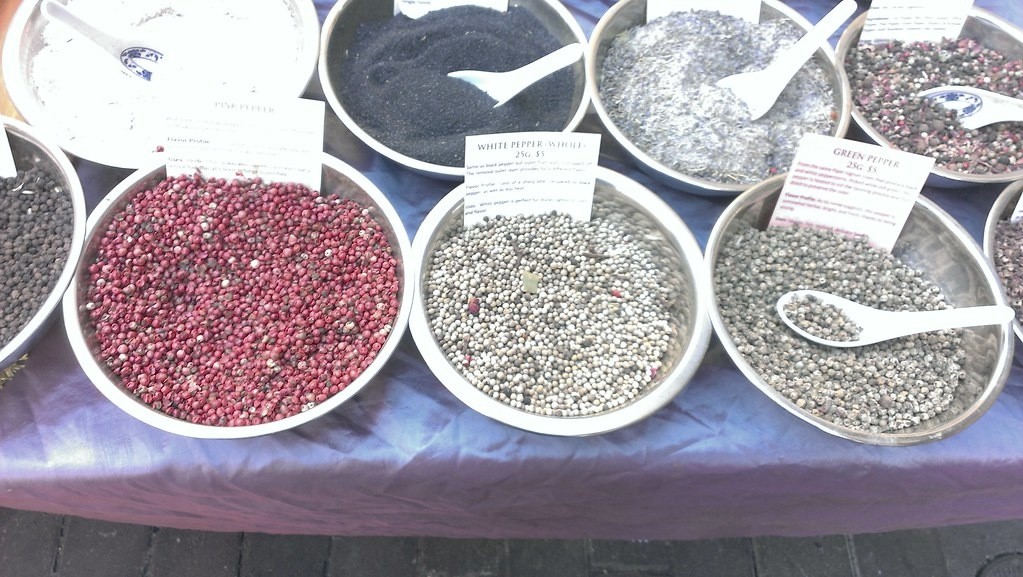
top-left (1, 0), bottom-right (1023, 542)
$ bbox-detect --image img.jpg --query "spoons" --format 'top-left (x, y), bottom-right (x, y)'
top-left (776, 289), bottom-right (1015, 349)
top-left (917, 86), bottom-right (1023, 131)
top-left (41, 0), bottom-right (165, 83)
top-left (449, 43), bottom-right (583, 108)
top-left (717, 0), bottom-right (858, 121)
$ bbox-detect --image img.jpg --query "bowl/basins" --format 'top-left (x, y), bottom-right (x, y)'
top-left (2, 0), bottom-right (320, 168)
top-left (319, 0), bottom-right (592, 181)
top-left (63, 152), bottom-right (415, 439)
top-left (982, 178), bottom-right (1023, 366)
top-left (0, 114), bottom-right (86, 369)
top-left (704, 172), bottom-right (1015, 447)
top-left (836, 5), bottom-right (1023, 188)
top-left (409, 165), bottom-right (713, 438)
top-left (589, 0), bottom-right (852, 196)
